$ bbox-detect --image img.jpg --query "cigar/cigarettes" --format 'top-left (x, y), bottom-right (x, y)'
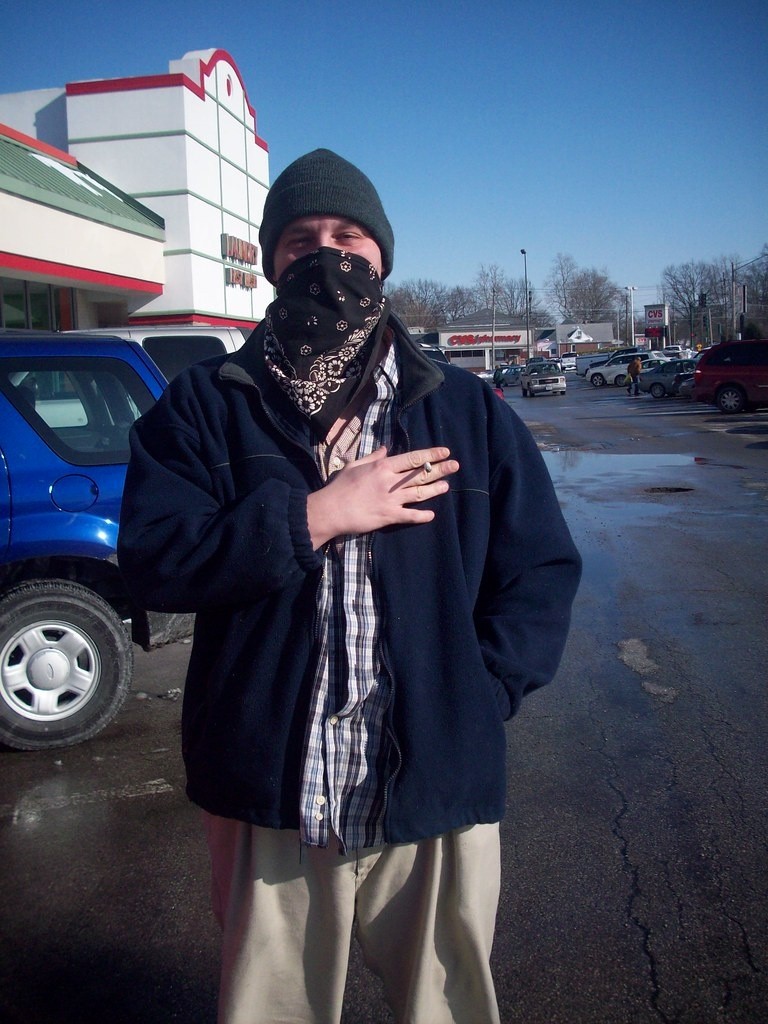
top-left (423, 461), bottom-right (432, 473)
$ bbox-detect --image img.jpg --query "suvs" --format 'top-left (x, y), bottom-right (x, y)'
top-left (688, 339), bottom-right (768, 413)
top-left (582, 351), bottom-right (670, 389)
top-left (0, 333), bottom-right (211, 753)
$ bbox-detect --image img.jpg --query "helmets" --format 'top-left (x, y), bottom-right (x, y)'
top-left (696, 344), bottom-right (702, 348)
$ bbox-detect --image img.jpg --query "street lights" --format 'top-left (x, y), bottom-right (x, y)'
top-left (625, 285), bottom-right (638, 347)
top-left (521, 248), bottom-right (530, 365)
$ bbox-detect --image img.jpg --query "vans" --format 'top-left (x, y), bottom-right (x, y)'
top-left (28, 325), bottom-right (246, 445)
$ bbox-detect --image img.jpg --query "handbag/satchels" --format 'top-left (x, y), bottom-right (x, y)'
top-left (624, 373), bottom-right (633, 383)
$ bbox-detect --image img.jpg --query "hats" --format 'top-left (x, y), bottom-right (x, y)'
top-left (258, 149), bottom-right (395, 286)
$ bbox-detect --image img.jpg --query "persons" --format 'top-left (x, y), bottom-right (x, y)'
top-left (117, 148), bottom-right (583, 1024)
top-left (627, 357), bottom-right (642, 396)
top-left (493, 365), bottom-right (504, 392)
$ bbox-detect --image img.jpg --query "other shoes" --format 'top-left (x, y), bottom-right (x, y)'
top-left (626, 388), bottom-right (630, 394)
top-left (635, 393), bottom-right (641, 395)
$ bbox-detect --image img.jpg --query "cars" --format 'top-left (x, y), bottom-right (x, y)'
top-left (491, 344), bottom-right (717, 389)
top-left (637, 358), bottom-right (699, 398)
top-left (519, 360), bottom-right (566, 399)
